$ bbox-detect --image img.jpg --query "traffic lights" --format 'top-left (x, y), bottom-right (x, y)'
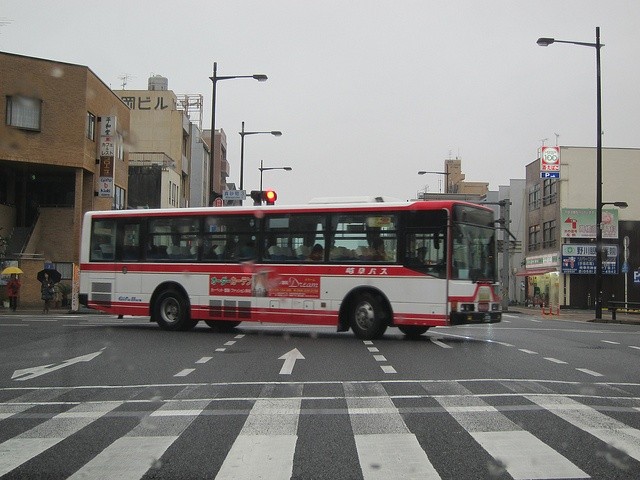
top-left (251, 190), bottom-right (277, 203)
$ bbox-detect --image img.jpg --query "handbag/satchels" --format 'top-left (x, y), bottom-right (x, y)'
top-left (42, 289), bottom-right (53, 300)
top-left (11, 284), bottom-right (17, 294)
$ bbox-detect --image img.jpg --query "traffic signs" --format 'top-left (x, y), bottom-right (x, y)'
top-left (222, 189), bottom-right (246, 200)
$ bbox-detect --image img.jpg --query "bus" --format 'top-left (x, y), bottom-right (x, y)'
top-left (78, 200), bottom-right (505, 340)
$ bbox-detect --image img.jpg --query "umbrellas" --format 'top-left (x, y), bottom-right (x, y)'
top-left (1, 267), bottom-right (24, 275)
top-left (36, 269), bottom-right (62, 283)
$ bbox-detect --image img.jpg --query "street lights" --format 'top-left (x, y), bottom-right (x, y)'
top-left (208, 62), bottom-right (267, 205)
top-left (418, 163), bottom-right (451, 192)
top-left (239, 122), bottom-right (282, 190)
top-left (537, 26), bottom-right (629, 317)
top-left (258, 160), bottom-right (292, 191)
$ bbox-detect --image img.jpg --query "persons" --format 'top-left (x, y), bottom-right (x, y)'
top-left (373, 240), bottom-right (394, 262)
top-left (7, 274), bottom-right (21, 312)
top-left (41, 272), bottom-right (54, 315)
top-left (411, 246), bottom-right (430, 273)
top-left (309, 243), bottom-right (324, 261)
